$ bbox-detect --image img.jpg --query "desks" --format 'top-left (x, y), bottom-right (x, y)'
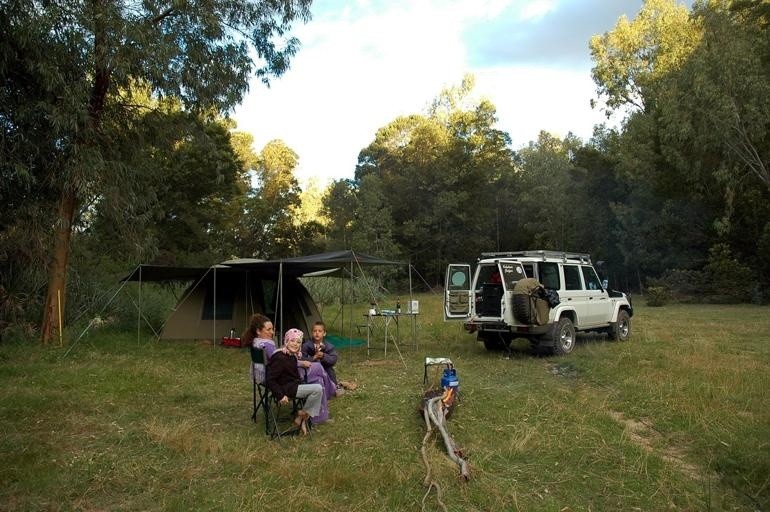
top-left (363, 312), bottom-right (420, 358)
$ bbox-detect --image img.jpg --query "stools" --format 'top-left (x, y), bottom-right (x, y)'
top-left (423, 356), bottom-right (453, 386)
top-left (267, 394), bottom-right (313, 442)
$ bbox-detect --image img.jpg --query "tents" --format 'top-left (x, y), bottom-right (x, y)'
top-left (159, 266), bottom-right (326, 340)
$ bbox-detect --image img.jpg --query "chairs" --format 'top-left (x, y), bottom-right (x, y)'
top-left (251, 343), bottom-right (308, 429)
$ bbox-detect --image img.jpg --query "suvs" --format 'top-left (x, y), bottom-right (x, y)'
top-left (438, 245), bottom-right (637, 356)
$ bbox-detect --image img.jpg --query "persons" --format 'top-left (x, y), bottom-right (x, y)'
top-left (300, 321), bottom-right (340, 386)
top-left (248, 313), bottom-right (347, 427)
top-left (269, 328), bottom-right (324, 436)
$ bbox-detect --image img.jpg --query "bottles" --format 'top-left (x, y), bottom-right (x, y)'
top-left (230, 327), bottom-right (236, 339)
top-left (396, 296), bottom-right (401, 313)
